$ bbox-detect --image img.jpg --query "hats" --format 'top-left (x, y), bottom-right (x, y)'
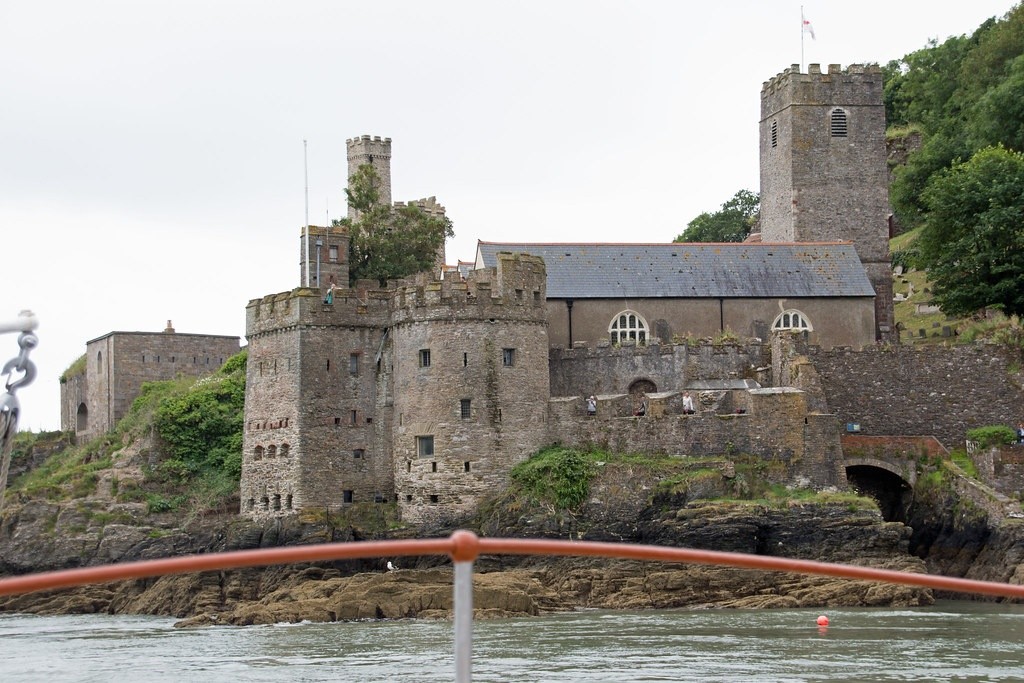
top-left (589, 395), bottom-right (594, 400)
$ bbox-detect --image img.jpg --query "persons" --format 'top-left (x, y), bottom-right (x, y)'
top-left (588, 396), bottom-right (597, 415)
top-left (683, 393), bottom-right (693, 413)
top-left (327, 284), bottom-right (335, 304)
top-left (637, 398), bottom-right (646, 416)
top-left (1018, 424), bottom-right (1024, 442)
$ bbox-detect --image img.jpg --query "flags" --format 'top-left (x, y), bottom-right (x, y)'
top-left (803, 13), bottom-right (815, 40)
